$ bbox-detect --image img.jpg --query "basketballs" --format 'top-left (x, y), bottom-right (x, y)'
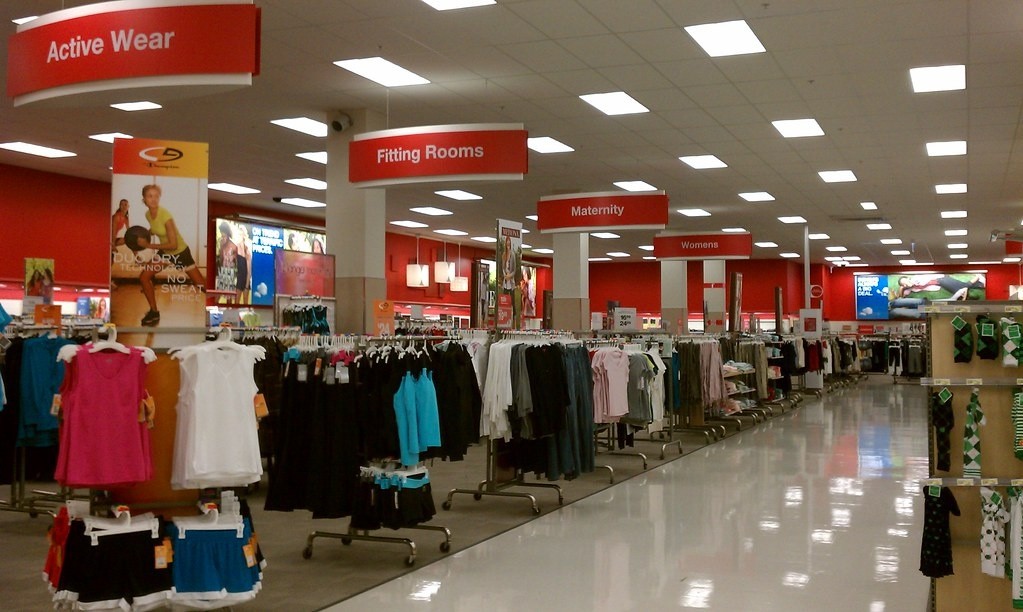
top-left (126, 226), bottom-right (151, 251)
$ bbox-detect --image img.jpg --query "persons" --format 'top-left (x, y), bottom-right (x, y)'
top-left (39, 268), bottom-right (53, 303)
top-left (521, 267), bottom-right (535, 317)
top-left (138, 185), bottom-right (206, 324)
top-left (502, 237), bottom-right (516, 330)
top-left (27, 270), bottom-right (43, 296)
top-left (899, 274), bottom-right (986, 301)
top-left (888, 287), bottom-right (968, 320)
top-left (312, 239), bottom-right (324, 254)
top-left (95, 299), bottom-right (107, 318)
top-left (235, 225), bottom-right (251, 304)
top-left (481, 269), bottom-right (489, 327)
top-left (111, 199), bottom-right (142, 291)
top-left (288, 234), bottom-right (300, 251)
top-left (216, 222), bottom-right (237, 304)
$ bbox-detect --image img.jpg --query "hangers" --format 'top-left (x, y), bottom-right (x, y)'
top-left (267, 320), bottom-right (491, 368)
top-left (360, 459), bottom-right (429, 481)
top-left (283, 294), bottom-right (333, 314)
top-left (908, 334), bottom-right (921, 342)
top-left (831, 331), bottom-right (857, 342)
top-left (83, 490), bottom-right (245, 547)
top-left (6, 318), bottom-right (158, 365)
top-left (165, 325), bottom-right (267, 363)
top-left (584, 333), bottom-right (642, 355)
top-left (494, 329), bottom-right (583, 349)
top-left (860, 332), bottom-right (886, 342)
top-left (647, 332), bottom-right (722, 345)
top-left (784, 335), bottom-right (804, 342)
top-left (735, 337), bottom-right (764, 346)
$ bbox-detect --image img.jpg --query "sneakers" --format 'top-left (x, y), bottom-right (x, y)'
top-left (142, 308), bottom-right (160, 327)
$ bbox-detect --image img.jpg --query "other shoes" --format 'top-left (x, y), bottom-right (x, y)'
top-left (961, 287), bottom-right (968, 301)
top-left (976, 274), bottom-right (986, 283)
